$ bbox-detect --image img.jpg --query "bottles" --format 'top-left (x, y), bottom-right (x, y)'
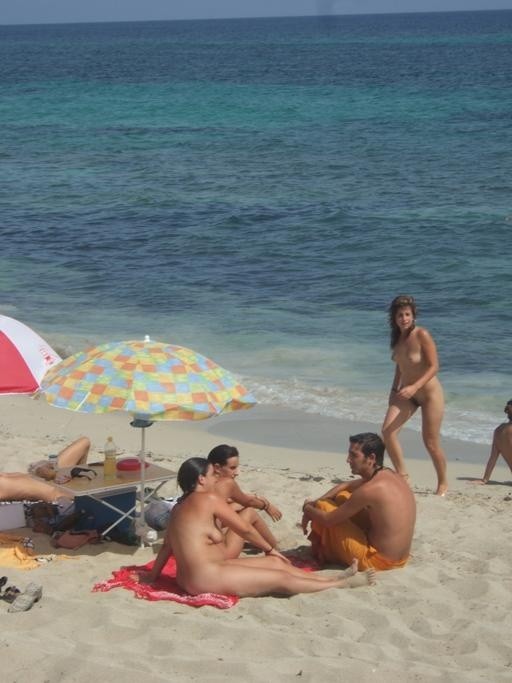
top-left (103, 435), bottom-right (117, 479)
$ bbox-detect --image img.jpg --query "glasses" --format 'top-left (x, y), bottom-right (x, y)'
top-left (71, 467), bottom-right (97, 481)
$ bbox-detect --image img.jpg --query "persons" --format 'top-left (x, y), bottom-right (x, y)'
top-left (1, 436), bottom-right (91, 502)
top-left (206, 444), bottom-right (281, 559)
top-left (381, 294), bottom-right (449, 497)
top-left (130, 457), bottom-right (377, 597)
top-left (466, 400), bottom-right (512, 485)
top-left (300, 431), bottom-right (416, 570)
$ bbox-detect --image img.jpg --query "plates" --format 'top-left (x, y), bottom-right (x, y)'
top-left (117, 458), bottom-right (151, 469)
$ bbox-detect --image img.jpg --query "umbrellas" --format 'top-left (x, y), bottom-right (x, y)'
top-left (40, 334), bottom-right (259, 550)
top-left (0, 312), bottom-right (65, 395)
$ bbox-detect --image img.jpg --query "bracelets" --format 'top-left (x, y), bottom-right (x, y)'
top-left (265, 546), bottom-right (274, 553)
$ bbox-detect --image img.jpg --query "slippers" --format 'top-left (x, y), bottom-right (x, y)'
top-left (136, 491), bottom-right (156, 504)
top-left (145, 486), bottom-right (164, 502)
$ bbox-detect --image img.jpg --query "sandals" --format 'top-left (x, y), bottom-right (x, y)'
top-left (0, 576), bottom-right (43, 614)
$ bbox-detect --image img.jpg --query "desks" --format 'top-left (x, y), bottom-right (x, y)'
top-left (35, 453), bottom-right (178, 544)
